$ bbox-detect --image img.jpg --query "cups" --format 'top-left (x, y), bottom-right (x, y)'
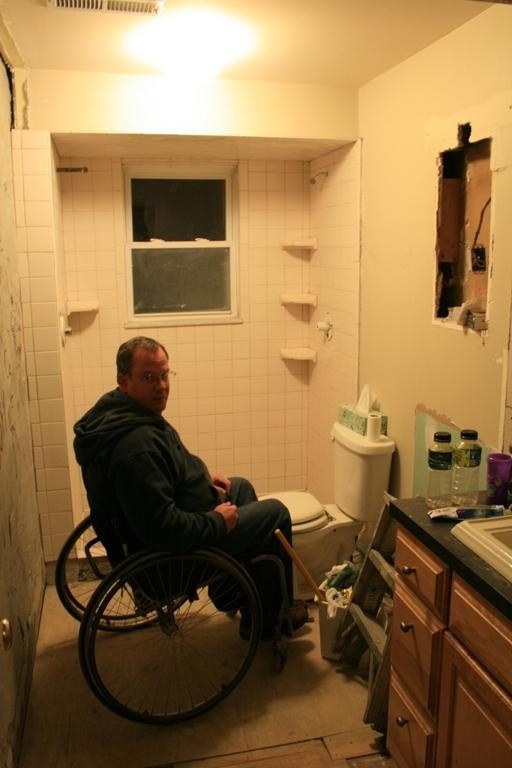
top-left (487, 450), bottom-right (510, 510)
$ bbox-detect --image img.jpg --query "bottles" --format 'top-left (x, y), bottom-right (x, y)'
top-left (426, 430), bottom-right (481, 514)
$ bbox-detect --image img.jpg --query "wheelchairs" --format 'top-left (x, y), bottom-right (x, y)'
top-left (55, 514), bottom-right (288, 727)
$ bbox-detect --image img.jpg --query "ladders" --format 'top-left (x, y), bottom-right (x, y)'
top-left (333, 491), bottom-right (405, 720)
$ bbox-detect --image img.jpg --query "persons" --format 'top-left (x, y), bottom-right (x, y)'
top-left (73, 336), bottom-right (309, 642)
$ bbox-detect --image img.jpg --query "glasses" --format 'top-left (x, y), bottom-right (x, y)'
top-left (129, 368), bottom-right (171, 385)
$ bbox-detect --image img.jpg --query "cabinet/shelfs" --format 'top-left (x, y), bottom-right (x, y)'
top-left (382, 520), bottom-right (450, 766)
top-left (432, 570), bottom-right (512, 766)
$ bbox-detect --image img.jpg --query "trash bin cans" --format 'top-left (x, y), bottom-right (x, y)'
top-left (314, 575), bottom-right (353, 662)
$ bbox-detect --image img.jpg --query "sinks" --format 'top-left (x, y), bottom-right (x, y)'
top-left (452, 514), bottom-right (512, 581)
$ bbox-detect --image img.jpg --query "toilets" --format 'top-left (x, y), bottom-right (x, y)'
top-left (255, 421), bottom-right (396, 601)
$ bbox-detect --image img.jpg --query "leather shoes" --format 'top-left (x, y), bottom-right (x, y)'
top-left (239, 600), bottom-right (308, 641)
